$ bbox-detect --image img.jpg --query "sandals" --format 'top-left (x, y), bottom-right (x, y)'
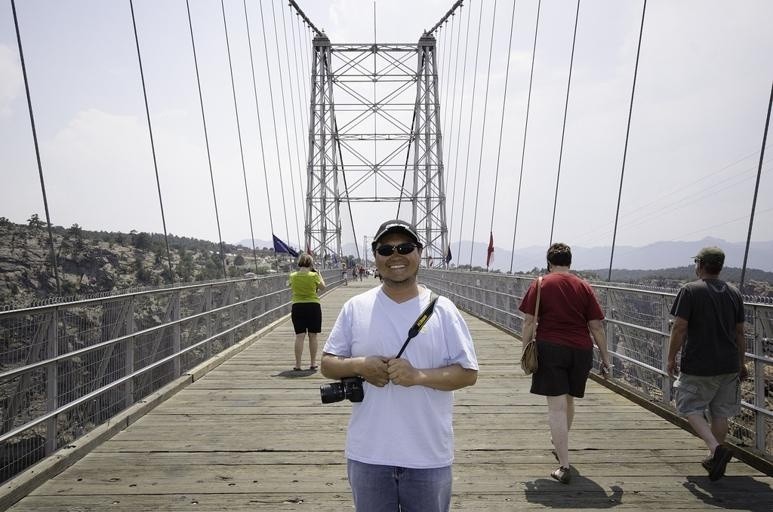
top-left (551, 466), bottom-right (570, 484)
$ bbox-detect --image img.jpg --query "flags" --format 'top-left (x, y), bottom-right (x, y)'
top-left (428, 256), bottom-right (433, 266)
top-left (272, 235), bottom-right (298, 257)
top-left (445, 245), bottom-right (453, 264)
top-left (486, 233), bottom-right (496, 268)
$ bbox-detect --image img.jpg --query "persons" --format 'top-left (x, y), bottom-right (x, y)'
top-left (517, 238), bottom-right (614, 485)
top-left (351, 264), bottom-right (364, 281)
top-left (320, 220), bottom-right (478, 510)
top-left (286, 252), bottom-right (326, 371)
top-left (664, 244), bottom-right (749, 484)
top-left (340, 262), bottom-right (348, 285)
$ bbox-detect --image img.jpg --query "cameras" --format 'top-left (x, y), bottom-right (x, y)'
top-left (318, 376), bottom-right (367, 405)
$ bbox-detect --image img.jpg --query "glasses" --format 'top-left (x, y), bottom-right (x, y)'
top-left (695, 259), bottom-right (700, 263)
top-left (376, 245), bottom-right (414, 256)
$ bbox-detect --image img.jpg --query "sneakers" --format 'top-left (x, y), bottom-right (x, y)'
top-left (701, 444), bottom-right (734, 481)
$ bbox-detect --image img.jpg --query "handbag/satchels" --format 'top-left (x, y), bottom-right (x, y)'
top-left (520, 341), bottom-right (539, 375)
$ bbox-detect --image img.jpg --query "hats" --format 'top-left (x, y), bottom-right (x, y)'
top-left (690, 246), bottom-right (725, 263)
top-left (372, 220), bottom-right (422, 246)
